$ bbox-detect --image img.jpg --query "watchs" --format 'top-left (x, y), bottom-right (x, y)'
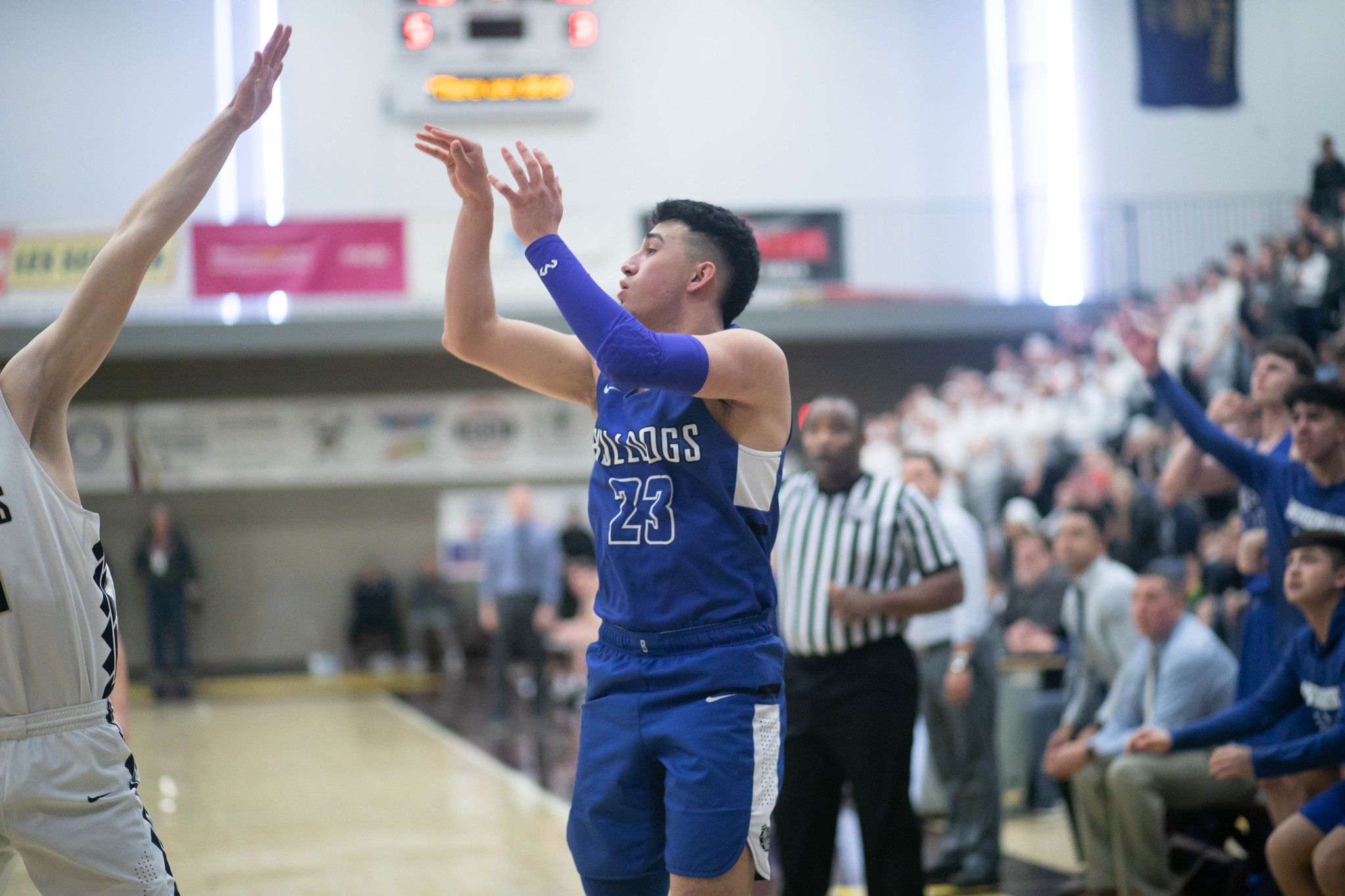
top-left (948, 654), bottom-right (972, 672)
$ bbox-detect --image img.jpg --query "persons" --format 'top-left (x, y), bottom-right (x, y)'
top-left (343, 124), bottom-right (1345, 896)
top-left (0, 24), bottom-right (295, 896)
top-left (132, 502), bottom-right (198, 699)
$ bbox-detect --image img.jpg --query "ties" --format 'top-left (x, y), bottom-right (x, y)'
top-left (1140, 635), bottom-right (1160, 732)
top-left (1075, 584), bottom-right (1092, 644)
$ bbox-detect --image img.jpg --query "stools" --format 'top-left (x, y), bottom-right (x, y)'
top-left (1167, 804), bottom-right (1261, 896)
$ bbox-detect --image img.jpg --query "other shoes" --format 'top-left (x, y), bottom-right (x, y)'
top-left (951, 858), bottom-right (1006, 889)
top-left (923, 852), bottom-right (959, 884)
